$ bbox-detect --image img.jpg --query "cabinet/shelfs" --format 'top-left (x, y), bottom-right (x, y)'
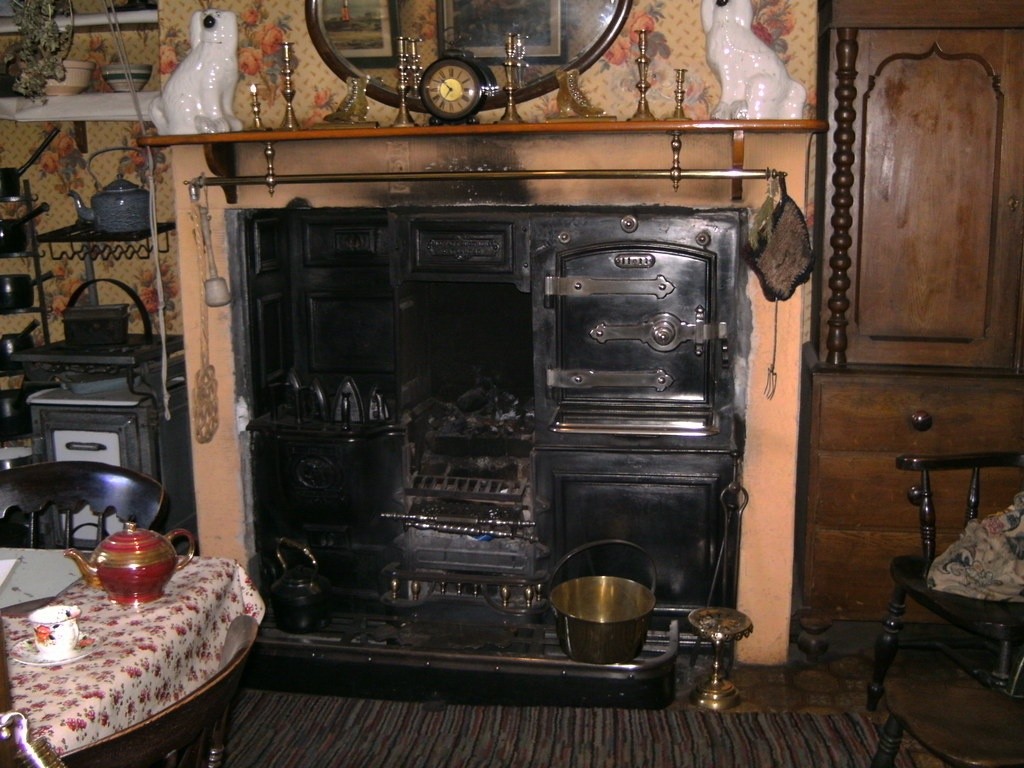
top-left (0, 0), bottom-right (184, 408)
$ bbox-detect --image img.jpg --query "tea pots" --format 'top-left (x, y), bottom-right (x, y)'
top-left (63, 521), bottom-right (195, 608)
top-left (67, 146), bottom-right (155, 232)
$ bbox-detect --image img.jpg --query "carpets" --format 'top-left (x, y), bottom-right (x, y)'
top-left (219, 687), bottom-right (923, 768)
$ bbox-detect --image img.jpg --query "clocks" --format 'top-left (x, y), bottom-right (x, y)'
top-left (417, 26), bottom-right (487, 126)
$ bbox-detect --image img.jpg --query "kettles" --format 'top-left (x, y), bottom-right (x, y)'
top-left (262, 538), bottom-right (333, 634)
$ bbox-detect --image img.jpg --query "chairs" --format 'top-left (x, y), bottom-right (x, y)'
top-left (864, 451), bottom-right (1024, 710)
top-left (0, 460), bottom-right (166, 550)
top-left (869, 679), bottom-right (1024, 768)
top-left (0, 615), bottom-right (259, 768)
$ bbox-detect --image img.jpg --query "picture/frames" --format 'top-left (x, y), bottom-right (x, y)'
top-left (436, 0), bottom-right (568, 65)
top-left (317, 0), bottom-right (397, 68)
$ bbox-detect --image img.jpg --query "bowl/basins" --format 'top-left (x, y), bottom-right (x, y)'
top-left (0, 319), bottom-right (40, 371)
top-left (17, 61), bottom-right (96, 95)
top-left (100, 65), bottom-right (153, 92)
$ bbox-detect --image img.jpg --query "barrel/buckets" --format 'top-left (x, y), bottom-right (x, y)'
top-left (546, 539), bottom-right (658, 664)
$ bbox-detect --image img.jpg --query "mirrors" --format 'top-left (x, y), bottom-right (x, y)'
top-left (303, 0), bottom-right (634, 111)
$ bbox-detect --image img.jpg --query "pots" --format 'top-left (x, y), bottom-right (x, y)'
top-left (0, 126), bottom-right (61, 198)
top-left (0, 271), bottom-right (55, 313)
top-left (0, 202), bottom-right (50, 253)
top-left (0, 383), bottom-right (61, 434)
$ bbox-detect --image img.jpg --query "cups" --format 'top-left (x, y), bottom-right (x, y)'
top-left (27, 605), bottom-right (82, 658)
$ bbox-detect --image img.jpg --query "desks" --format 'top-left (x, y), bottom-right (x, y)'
top-left (0, 547), bottom-right (267, 768)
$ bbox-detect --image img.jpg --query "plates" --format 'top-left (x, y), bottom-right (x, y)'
top-left (9, 632), bottom-right (100, 667)
